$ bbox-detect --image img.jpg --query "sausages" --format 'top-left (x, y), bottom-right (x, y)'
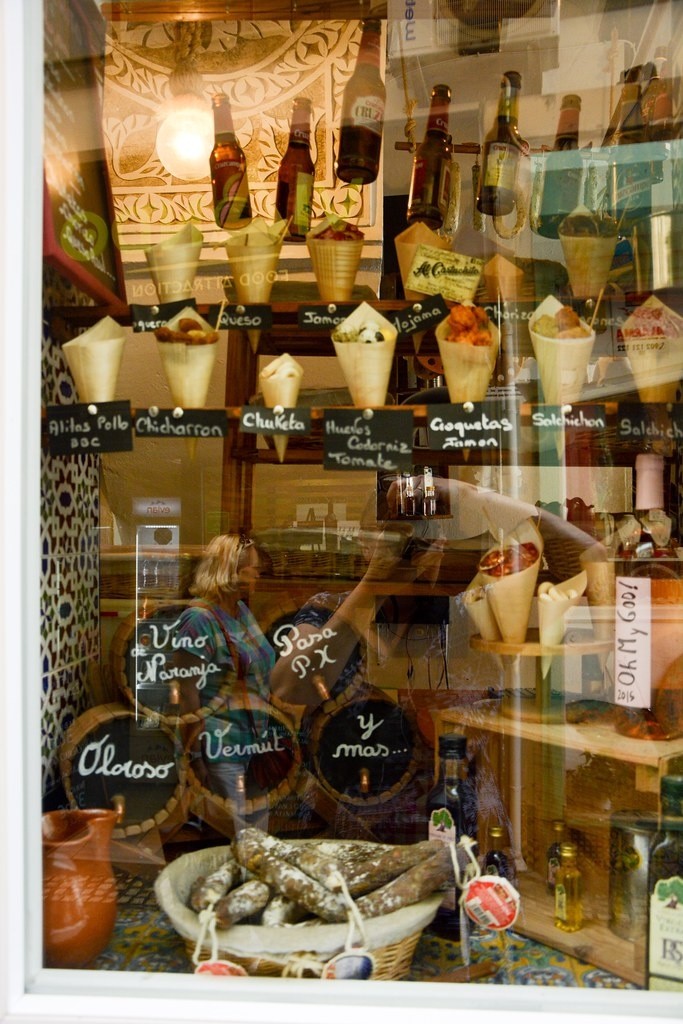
top-left (189, 827), bottom-right (470, 927)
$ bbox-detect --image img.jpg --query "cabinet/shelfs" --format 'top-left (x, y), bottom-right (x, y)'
top-left (99, 299), bottom-right (683, 599)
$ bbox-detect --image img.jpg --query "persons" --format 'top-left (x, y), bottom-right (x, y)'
top-left (267, 473), bottom-right (608, 848)
top-left (173, 535), bottom-right (281, 842)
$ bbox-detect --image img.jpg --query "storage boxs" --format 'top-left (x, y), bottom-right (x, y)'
top-left (432, 703), bottom-right (683, 988)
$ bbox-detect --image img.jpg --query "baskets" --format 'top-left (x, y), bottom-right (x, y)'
top-left (251, 519), bottom-right (414, 579)
top-left (98, 546), bottom-right (201, 599)
top-left (543, 531), bottom-right (598, 581)
top-left (157, 840), bottom-right (446, 980)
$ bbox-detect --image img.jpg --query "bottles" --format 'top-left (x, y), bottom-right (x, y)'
top-left (646, 777), bottom-right (683, 991)
top-left (474, 70), bottom-right (523, 216)
top-left (553, 841), bottom-right (584, 932)
top-left (333, 17), bottom-right (389, 184)
top-left (544, 821), bottom-right (568, 896)
top-left (480, 826), bottom-right (512, 882)
top-left (208, 93), bottom-right (254, 230)
top-left (616, 452), bottom-right (683, 739)
top-left (274, 97), bottom-right (315, 242)
top-left (406, 84), bottom-right (454, 232)
top-left (41, 807), bottom-right (119, 971)
top-left (607, 85), bottom-right (652, 230)
top-left (536, 95), bottom-right (584, 238)
top-left (424, 732), bottom-right (478, 846)
